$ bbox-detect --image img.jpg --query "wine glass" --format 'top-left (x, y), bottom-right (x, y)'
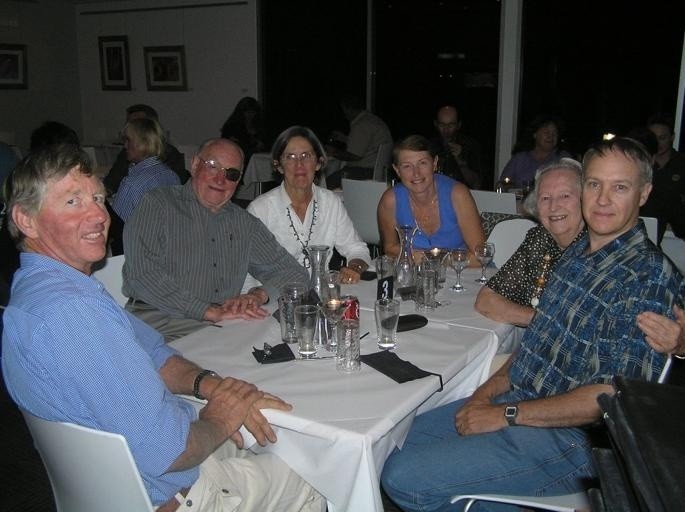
top-left (449, 248), bottom-right (468, 292)
top-left (474, 242), bottom-right (495, 283)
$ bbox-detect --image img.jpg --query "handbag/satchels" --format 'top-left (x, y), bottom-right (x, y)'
top-left (588, 374), bottom-right (685, 511)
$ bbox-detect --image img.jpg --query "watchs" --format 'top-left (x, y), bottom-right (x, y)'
top-left (503, 399), bottom-right (522, 427)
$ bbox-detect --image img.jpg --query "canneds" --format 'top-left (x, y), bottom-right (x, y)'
top-left (342, 294), bottom-right (360, 321)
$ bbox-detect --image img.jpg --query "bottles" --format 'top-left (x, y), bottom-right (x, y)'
top-left (302, 245), bottom-right (331, 306)
top-left (391, 224), bottom-right (419, 295)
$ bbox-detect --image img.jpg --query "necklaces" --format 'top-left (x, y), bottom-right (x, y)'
top-left (412, 194), bottom-right (436, 237)
top-left (287, 199), bottom-right (318, 254)
top-left (530, 239), bottom-right (553, 308)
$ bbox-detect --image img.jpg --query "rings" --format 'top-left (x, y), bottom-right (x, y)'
top-left (348, 278), bottom-right (352, 281)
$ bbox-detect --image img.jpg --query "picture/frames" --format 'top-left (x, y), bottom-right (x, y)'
top-left (98, 34), bottom-right (131, 91)
top-left (0, 43), bottom-right (27, 89)
top-left (142, 45), bottom-right (189, 92)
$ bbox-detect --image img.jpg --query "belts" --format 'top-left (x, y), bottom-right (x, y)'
top-left (153, 486), bottom-right (191, 512)
top-left (127, 296), bottom-right (145, 304)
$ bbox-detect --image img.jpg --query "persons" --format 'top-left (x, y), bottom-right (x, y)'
top-left (1, 140), bottom-right (330, 512)
top-left (494, 117), bottom-right (577, 200)
top-left (28, 119), bottom-right (80, 152)
top-left (218, 96), bottom-right (267, 186)
top-left (241, 122), bottom-right (376, 295)
top-left (104, 116), bottom-right (182, 257)
top-left (614, 124), bottom-right (669, 249)
top-left (473, 157), bottom-right (685, 380)
top-left (380, 133), bottom-right (488, 270)
top-left (324, 96), bottom-right (393, 190)
top-left (101, 103), bottom-right (192, 186)
top-left (648, 116), bottom-right (685, 240)
top-left (119, 137), bottom-right (312, 344)
top-left (378, 135), bottom-right (685, 512)
top-left (428, 105), bottom-right (487, 189)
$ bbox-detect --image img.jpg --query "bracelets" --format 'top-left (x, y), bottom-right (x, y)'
top-left (193, 370), bottom-right (216, 400)
top-left (674, 354), bottom-right (685, 360)
top-left (348, 262), bottom-right (362, 274)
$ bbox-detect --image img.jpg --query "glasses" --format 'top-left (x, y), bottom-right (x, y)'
top-left (280, 152), bottom-right (315, 162)
top-left (437, 122), bottom-right (455, 129)
top-left (198, 157), bottom-right (241, 182)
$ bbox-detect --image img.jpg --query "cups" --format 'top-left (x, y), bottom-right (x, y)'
top-left (285, 281), bottom-right (306, 305)
top-left (278, 295), bottom-right (299, 344)
top-left (294, 305), bottom-right (321, 358)
top-left (414, 270), bottom-right (436, 313)
top-left (373, 297), bottom-right (399, 350)
top-left (421, 248), bottom-right (448, 289)
top-left (336, 319), bottom-right (361, 372)
